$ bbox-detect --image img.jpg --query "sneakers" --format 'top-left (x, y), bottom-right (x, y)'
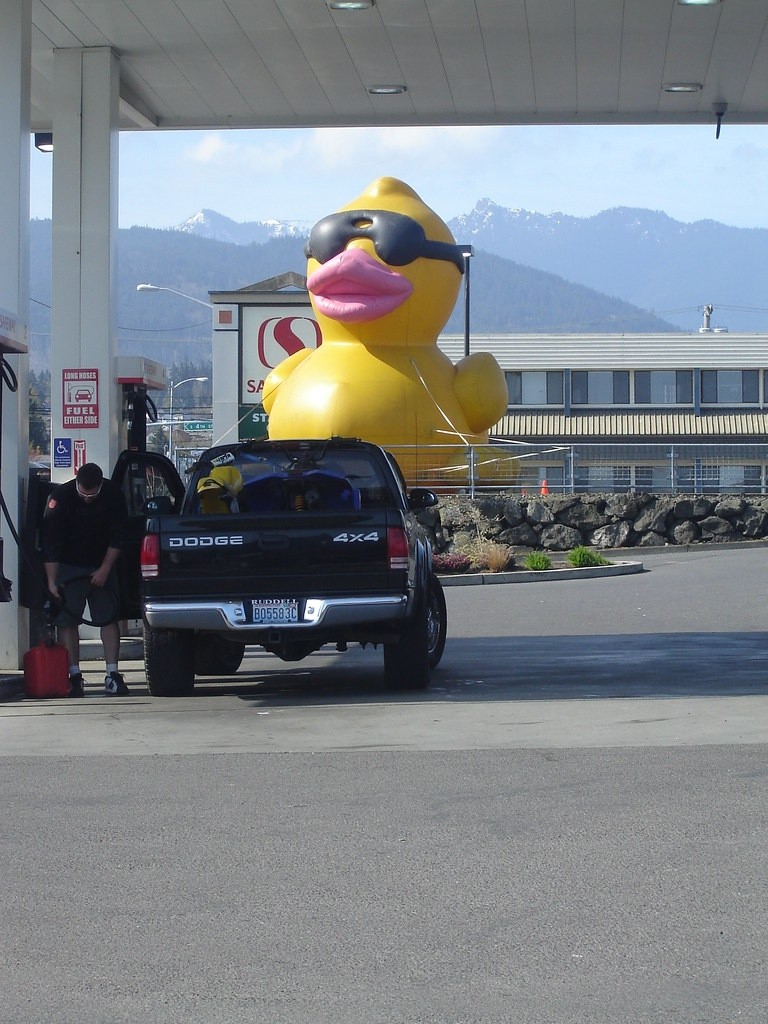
top-left (68, 673), bottom-right (86, 698)
top-left (105, 670), bottom-right (129, 694)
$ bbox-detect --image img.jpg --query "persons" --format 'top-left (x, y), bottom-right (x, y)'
top-left (38, 463), bottom-right (129, 698)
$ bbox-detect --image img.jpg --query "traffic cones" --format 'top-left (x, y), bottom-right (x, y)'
top-left (539, 480), bottom-right (550, 496)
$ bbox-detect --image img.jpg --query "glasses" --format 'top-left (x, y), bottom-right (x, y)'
top-left (76, 479), bottom-right (102, 499)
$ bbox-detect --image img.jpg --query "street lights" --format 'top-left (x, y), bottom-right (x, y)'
top-left (168, 373), bottom-right (209, 464)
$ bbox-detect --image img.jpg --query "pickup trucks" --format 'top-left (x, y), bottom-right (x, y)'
top-left (112, 435), bottom-right (448, 697)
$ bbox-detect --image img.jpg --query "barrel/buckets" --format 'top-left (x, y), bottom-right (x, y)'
top-left (24, 641), bottom-right (69, 699)
